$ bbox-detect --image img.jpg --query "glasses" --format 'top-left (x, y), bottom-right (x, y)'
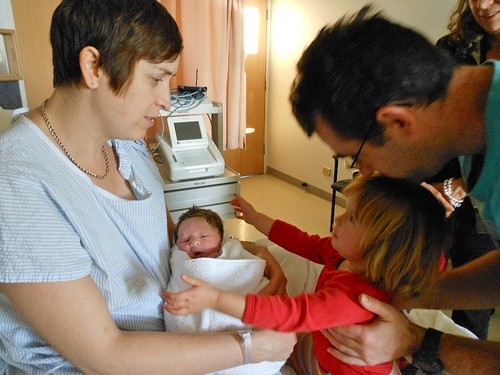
top-left (344, 141), bottom-right (366, 171)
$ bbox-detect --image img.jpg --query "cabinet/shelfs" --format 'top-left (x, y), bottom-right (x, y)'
top-left (157, 161), bottom-right (239, 223)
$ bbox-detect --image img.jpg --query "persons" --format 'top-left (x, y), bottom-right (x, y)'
top-left (163, 172), bottom-right (448, 375)
top-left (0, 0), bottom-right (298, 375)
top-left (289, 6), bottom-right (500, 375)
top-left (427, 0), bottom-right (500, 339)
top-left (171, 207), bottom-right (263, 375)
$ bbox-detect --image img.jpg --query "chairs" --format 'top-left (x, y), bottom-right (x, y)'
top-left (330, 153), bottom-right (356, 234)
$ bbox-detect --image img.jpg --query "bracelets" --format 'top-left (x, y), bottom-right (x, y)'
top-left (238, 329), bottom-right (252, 365)
top-left (443, 176), bottom-right (464, 209)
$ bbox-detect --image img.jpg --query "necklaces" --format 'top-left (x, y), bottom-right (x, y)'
top-left (41, 97), bottom-right (111, 180)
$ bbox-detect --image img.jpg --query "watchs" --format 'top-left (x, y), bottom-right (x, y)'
top-left (412, 327), bottom-right (444, 375)
